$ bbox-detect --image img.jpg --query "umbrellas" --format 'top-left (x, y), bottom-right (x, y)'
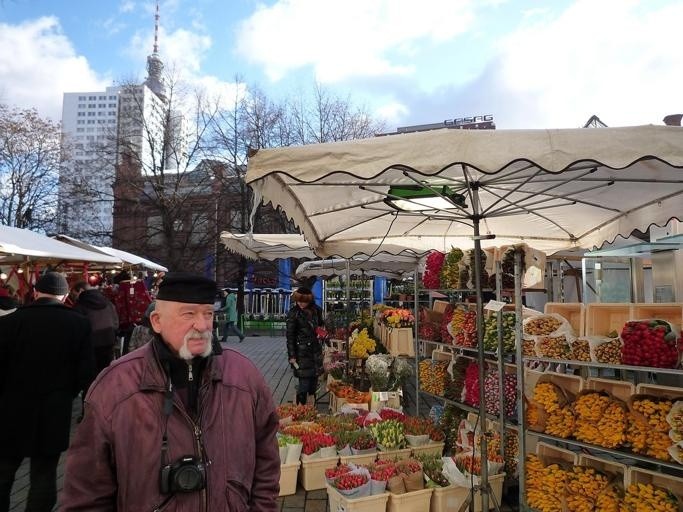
top-left (102, 245), bottom-right (169, 278)
top-left (290, 253), bottom-right (441, 380)
top-left (242, 128), bottom-right (682, 511)
top-left (54, 230), bottom-right (139, 277)
top-left (218, 229), bottom-right (446, 415)
top-left (0, 224), bottom-right (119, 280)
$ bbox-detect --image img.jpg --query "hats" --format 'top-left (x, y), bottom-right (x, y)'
top-left (35, 271), bottom-right (69, 294)
top-left (157, 272), bottom-right (217, 304)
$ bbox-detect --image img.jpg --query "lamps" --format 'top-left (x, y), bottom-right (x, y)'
top-left (383, 184), bottom-right (468, 212)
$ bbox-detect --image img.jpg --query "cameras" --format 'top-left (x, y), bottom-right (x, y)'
top-left (159, 454), bottom-right (205, 494)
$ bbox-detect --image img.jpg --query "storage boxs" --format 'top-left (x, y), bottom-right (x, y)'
top-left (278, 318), bottom-right (447, 512)
top-left (432, 242), bottom-right (683, 341)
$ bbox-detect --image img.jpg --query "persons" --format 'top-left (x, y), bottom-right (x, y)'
top-left (1, 271), bottom-right (281, 511)
top-left (286, 287), bottom-right (328, 408)
